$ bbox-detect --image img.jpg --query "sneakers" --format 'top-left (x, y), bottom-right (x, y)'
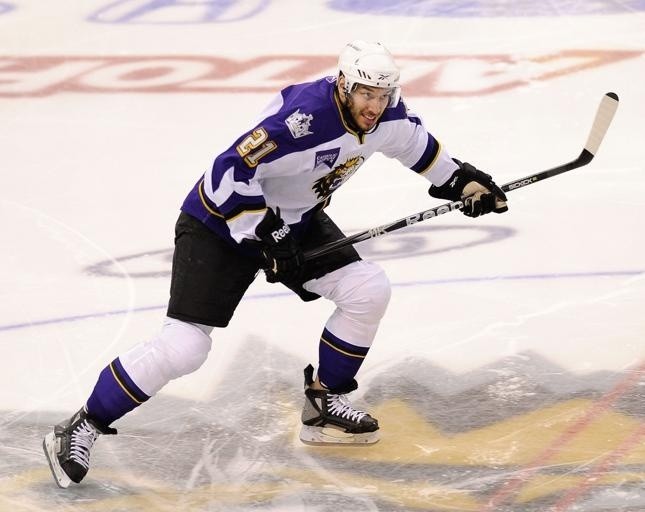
top-left (53, 405), bottom-right (118, 484)
top-left (301, 363), bottom-right (380, 435)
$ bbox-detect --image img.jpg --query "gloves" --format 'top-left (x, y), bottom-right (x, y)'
top-left (427, 156), bottom-right (509, 219)
top-left (241, 206), bottom-right (307, 284)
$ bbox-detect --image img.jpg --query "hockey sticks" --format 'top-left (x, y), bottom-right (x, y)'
top-left (302, 92), bottom-right (619, 262)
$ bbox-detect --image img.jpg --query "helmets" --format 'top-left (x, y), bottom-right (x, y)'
top-left (335, 38), bottom-right (402, 111)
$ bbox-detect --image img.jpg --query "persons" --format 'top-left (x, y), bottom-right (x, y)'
top-left (52, 36), bottom-right (509, 484)
